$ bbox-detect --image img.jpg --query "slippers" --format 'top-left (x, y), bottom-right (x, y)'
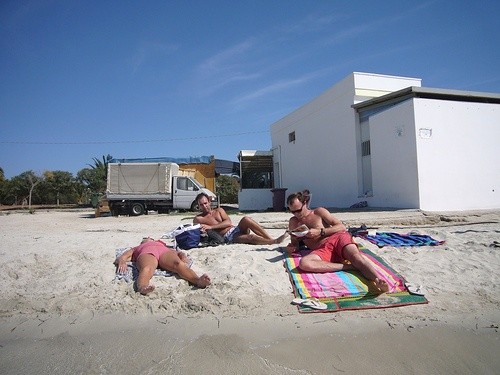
top-left (292, 297), bottom-right (327, 310)
top-left (404, 282), bottom-right (425, 296)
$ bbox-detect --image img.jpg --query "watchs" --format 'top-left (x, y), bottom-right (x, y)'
top-left (320, 228), bottom-right (325, 237)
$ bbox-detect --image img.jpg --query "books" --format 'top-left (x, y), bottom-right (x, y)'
top-left (286, 224), bottom-right (315, 250)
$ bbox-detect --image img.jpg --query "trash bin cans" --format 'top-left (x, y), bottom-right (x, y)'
top-left (91, 193), bottom-right (103, 208)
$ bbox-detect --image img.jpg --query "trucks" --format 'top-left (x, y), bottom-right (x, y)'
top-left (106, 161), bottom-right (218, 217)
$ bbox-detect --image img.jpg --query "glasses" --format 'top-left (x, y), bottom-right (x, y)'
top-left (291, 205), bottom-right (304, 214)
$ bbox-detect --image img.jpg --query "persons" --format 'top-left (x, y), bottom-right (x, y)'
top-left (194, 193), bottom-right (289, 244)
top-left (286, 193), bottom-right (389, 293)
top-left (115, 237), bottom-right (211, 297)
